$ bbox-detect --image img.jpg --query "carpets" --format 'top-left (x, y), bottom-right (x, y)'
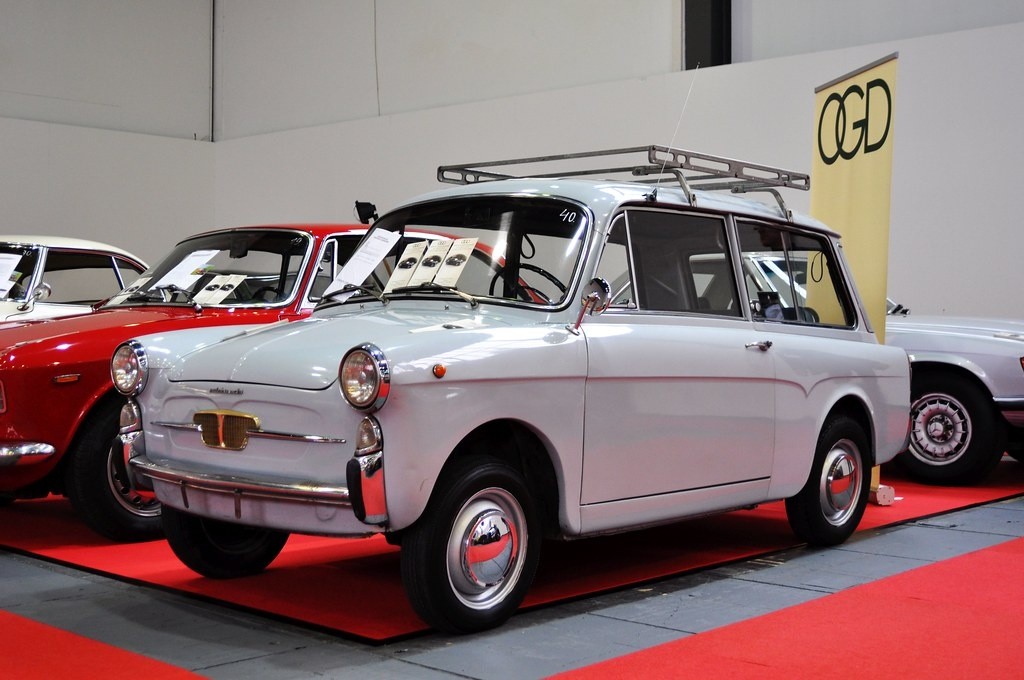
top-left (546, 538), bottom-right (1024, 679)
top-left (0, 610), bottom-right (202, 679)
top-left (2, 457), bottom-right (1023, 642)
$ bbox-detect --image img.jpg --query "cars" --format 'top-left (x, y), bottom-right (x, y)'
top-left (0, 222), bottom-right (551, 541)
top-left (599, 253), bottom-right (1024, 487)
top-left (107, 144), bottom-right (913, 637)
top-left (1, 235), bottom-right (171, 329)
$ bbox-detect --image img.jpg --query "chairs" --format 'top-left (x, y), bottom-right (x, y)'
top-left (641, 240), bottom-right (699, 310)
